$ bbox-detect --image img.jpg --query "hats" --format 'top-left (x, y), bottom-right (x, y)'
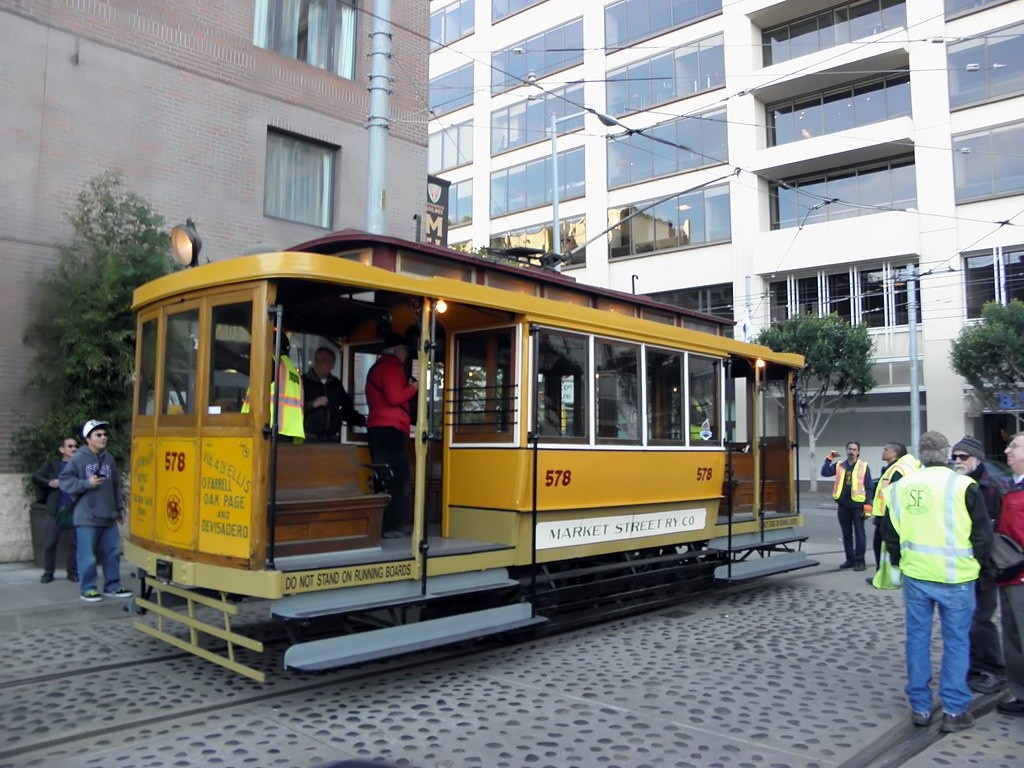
top-left (383, 333), bottom-right (412, 350)
top-left (83, 419), bottom-right (109, 438)
top-left (918, 431), bottom-right (951, 451)
top-left (953, 435), bottom-right (985, 461)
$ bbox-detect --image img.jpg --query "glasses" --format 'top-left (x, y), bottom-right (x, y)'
top-left (952, 454), bottom-right (973, 461)
top-left (69, 445), bottom-right (79, 448)
top-left (1006, 444), bottom-right (1024, 449)
top-left (96, 433), bottom-right (108, 437)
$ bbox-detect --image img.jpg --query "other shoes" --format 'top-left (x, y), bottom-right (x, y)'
top-left (866, 577), bottom-right (873, 585)
top-left (382, 528), bottom-right (412, 538)
top-left (839, 561), bottom-right (854, 568)
top-left (853, 560), bottom-right (866, 570)
top-left (41, 573), bottom-right (54, 582)
top-left (68, 572), bottom-right (80, 581)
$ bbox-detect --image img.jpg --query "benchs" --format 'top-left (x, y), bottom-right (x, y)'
top-left (266, 439), bottom-right (444, 559)
top-left (718, 452), bottom-right (782, 513)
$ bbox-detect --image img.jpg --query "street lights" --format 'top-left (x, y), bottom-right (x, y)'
top-left (552, 109), bottom-right (617, 271)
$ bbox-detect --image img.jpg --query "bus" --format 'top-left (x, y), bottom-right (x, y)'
top-left (123, 167), bottom-right (819, 682)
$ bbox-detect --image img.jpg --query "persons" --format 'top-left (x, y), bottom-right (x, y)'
top-left (996, 433), bottom-right (1024, 717)
top-left (33, 436), bottom-right (80, 584)
top-left (213, 328), bottom-right (306, 442)
top-left (952, 436), bottom-right (1005, 693)
top-left (302, 346), bottom-right (366, 441)
top-left (821, 442), bottom-right (874, 570)
top-left (677, 374), bottom-right (708, 440)
top-left (365, 338), bottom-right (417, 530)
top-left (58, 419), bottom-right (133, 601)
top-left (883, 431), bottom-right (993, 731)
top-left (866, 442), bottom-right (922, 584)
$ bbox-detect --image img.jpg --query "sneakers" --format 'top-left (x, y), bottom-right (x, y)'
top-left (913, 711), bottom-right (930, 726)
top-left (103, 587), bottom-right (133, 598)
top-left (80, 590), bottom-right (102, 602)
top-left (966, 669), bottom-right (976, 681)
top-left (969, 672), bottom-right (1010, 693)
top-left (941, 711), bottom-right (974, 731)
top-left (995, 698), bottom-right (1024, 716)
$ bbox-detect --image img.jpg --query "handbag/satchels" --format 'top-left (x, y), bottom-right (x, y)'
top-left (56, 502), bottom-right (74, 528)
top-left (410, 399), bottom-right (418, 425)
top-left (33, 478), bottom-right (46, 504)
top-left (989, 533), bottom-right (1024, 581)
